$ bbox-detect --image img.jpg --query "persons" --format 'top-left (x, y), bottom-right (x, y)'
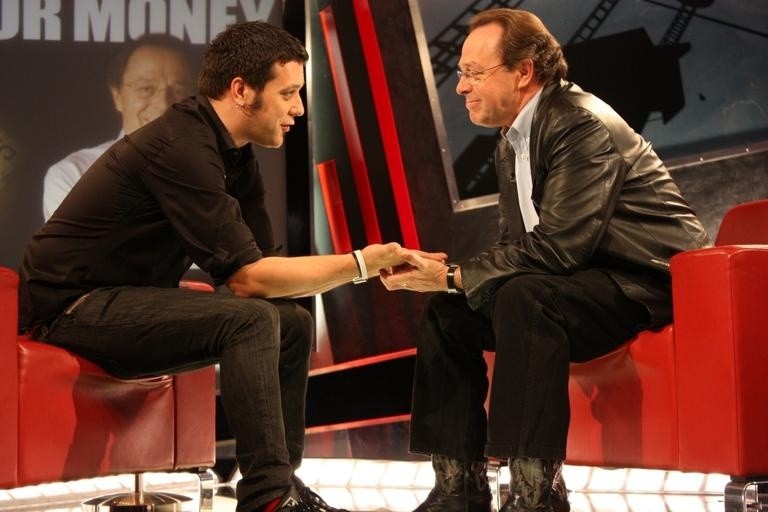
top-left (43, 35), bottom-right (198, 223)
top-left (379, 8), bottom-right (715, 512)
top-left (17, 21), bottom-right (448, 512)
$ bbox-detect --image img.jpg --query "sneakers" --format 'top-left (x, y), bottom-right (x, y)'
top-left (232, 473), bottom-right (349, 511)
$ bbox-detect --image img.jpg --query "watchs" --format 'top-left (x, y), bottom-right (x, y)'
top-left (447, 264), bottom-right (464, 294)
top-left (352, 249), bottom-right (369, 285)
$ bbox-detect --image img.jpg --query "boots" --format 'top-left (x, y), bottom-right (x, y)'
top-left (407, 454), bottom-right (494, 512)
top-left (493, 453), bottom-right (571, 511)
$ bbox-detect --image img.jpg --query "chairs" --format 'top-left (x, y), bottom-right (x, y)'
top-left (484, 198), bottom-right (768, 512)
top-left (0, 266), bottom-right (217, 512)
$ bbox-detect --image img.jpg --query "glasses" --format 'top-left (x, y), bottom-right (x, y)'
top-left (117, 78), bottom-right (193, 97)
top-left (454, 58), bottom-right (507, 78)
